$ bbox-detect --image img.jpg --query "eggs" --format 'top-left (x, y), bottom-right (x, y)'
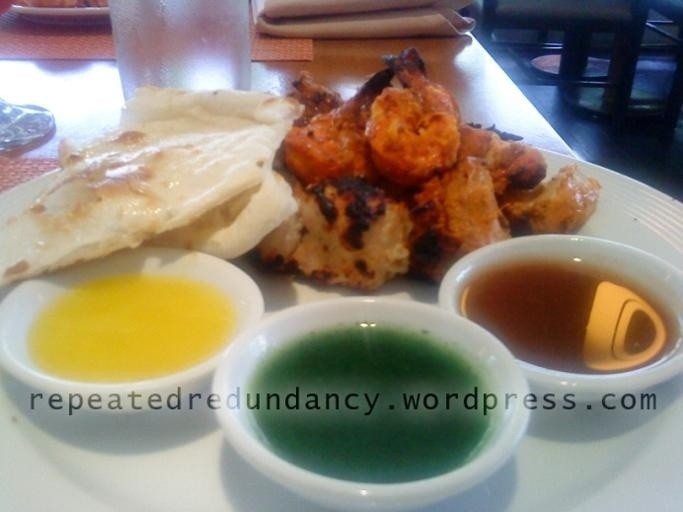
top-left (0, 85), bottom-right (307, 285)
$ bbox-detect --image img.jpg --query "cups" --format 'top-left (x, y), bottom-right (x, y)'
top-left (107, 0), bottom-right (251, 108)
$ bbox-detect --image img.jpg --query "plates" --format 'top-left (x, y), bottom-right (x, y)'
top-left (0, 136), bottom-right (681, 512)
top-left (434, 232), bottom-right (683, 408)
top-left (209, 294), bottom-right (533, 510)
top-left (0, 240), bottom-right (268, 409)
top-left (10, 3), bottom-right (111, 24)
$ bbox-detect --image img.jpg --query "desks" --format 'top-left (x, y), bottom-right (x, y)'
top-left (600, 1), bottom-right (682, 129)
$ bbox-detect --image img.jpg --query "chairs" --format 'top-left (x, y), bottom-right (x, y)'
top-left (480, 0), bottom-right (633, 118)
